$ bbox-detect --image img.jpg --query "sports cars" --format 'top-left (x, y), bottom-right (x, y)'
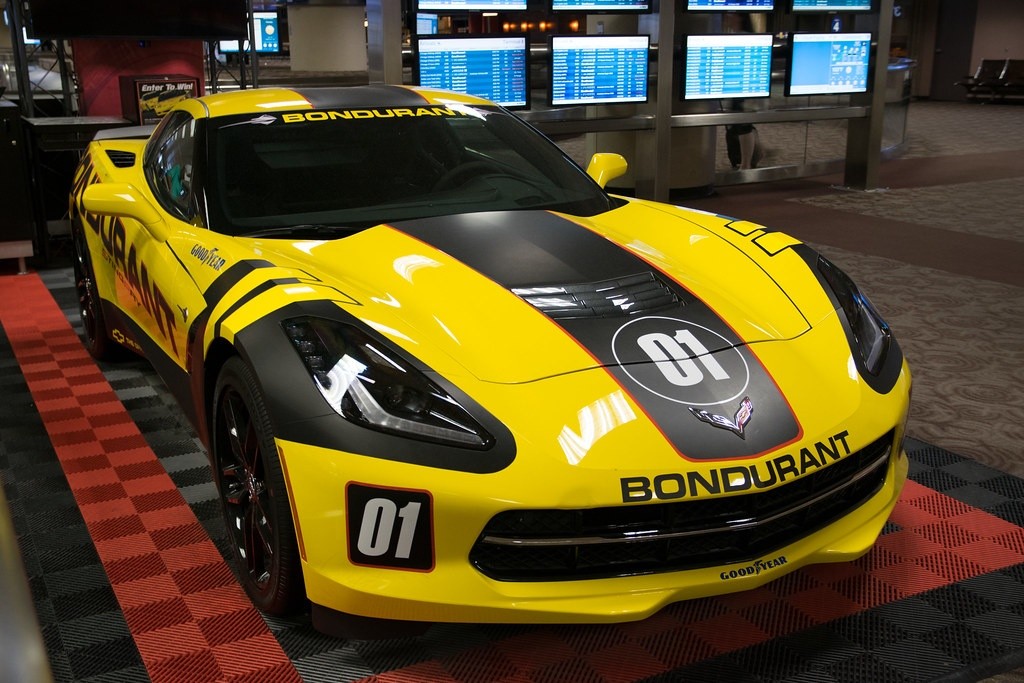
top-left (68, 87), bottom-right (914, 624)
top-left (152, 89), bottom-right (192, 116)
top-left (140, 90), bottom-right (159, 111)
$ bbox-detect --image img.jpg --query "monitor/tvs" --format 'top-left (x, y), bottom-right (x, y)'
top-left (218, 9), bottom-right (283, 54)
top-left (409, 0), bottom-right (872, 112)
top-left (19, 0), bottom-right (247, 41)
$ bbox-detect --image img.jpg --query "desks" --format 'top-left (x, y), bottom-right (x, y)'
top-left (21, 114), bottom-right (132, 266)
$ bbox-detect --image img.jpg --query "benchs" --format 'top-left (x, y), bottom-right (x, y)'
top-left (953, 59), bottom-right (1024, 105)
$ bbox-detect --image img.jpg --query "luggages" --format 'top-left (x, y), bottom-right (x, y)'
top-left (718, 98), bottom-right (762, 169)
